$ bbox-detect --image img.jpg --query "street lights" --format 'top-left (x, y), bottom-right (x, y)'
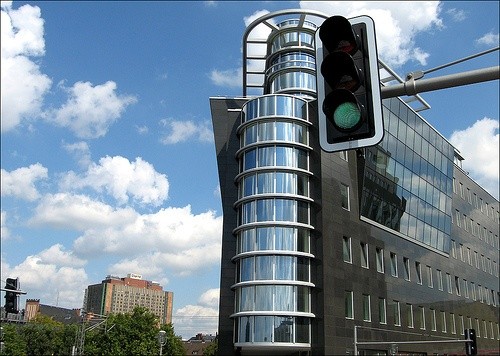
top-left (158, 330), bottom-right (167, 355)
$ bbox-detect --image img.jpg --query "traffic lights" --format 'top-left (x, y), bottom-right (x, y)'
top-left (312, 13), bottom-right (383, 152)
top-left (4, 277), bottom-right (20, 317)
top-left (464, 328), bottom-right (478, 355)
